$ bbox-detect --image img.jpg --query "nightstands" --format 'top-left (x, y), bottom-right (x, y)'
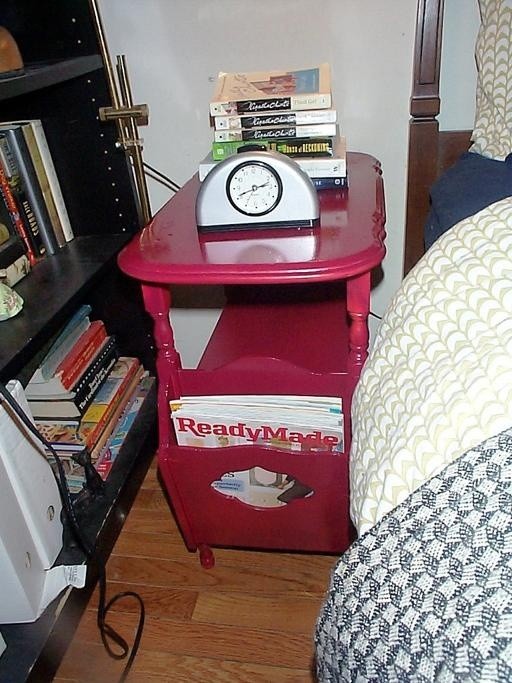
top-left (117, 152), bottom-right (387, 569)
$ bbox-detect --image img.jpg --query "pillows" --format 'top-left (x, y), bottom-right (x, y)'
top-left (467, 0), bottom-right (512, 162)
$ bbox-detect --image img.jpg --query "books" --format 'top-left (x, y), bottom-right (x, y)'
top-left (168, 396), bottom-right (344, 507)
top-left (0, 118), bottom-right (76, 289)
top-left (198, 62), bottom-right (347, 190)
top-left (24, 304), bottom-right (157, 494)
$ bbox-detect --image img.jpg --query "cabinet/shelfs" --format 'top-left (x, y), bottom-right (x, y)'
top-left (0, 0), bottom-right (157, 683)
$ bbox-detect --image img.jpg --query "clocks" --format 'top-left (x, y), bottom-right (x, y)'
top-left (196, 150), bottom-right (319, 235)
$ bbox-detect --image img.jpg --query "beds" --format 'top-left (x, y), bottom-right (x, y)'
top-left (312, 0), bottom-right (512, 683)
